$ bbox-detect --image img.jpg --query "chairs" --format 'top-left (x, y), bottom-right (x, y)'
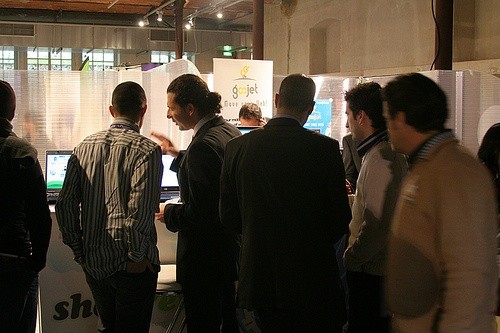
top-left (156, 265), bottom-right (186, 333)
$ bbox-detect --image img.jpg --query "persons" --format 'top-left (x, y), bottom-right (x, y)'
top-left (55, 81), bottom-right (163, 333)
top-left (0, 79), bottom-right (53, 333)
top-left (342, 72), bottom-right (500, 333)
top-left (151, 73), bottom-right (353, 333)
top-left (20, 110), bottom-right (59, 179)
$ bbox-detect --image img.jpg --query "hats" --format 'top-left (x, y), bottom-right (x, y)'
top-left (0, 80), bottom-right (16, 121)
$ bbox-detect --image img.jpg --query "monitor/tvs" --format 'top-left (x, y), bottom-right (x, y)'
top-left (45, 150), bottom-right (72, 194)
top-left (161, 153), bottom-right (180, 191)
top-left (237, 126), bottom-right (261, 135)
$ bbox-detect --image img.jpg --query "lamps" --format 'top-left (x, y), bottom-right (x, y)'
top-left (217, 9), bottom-right (224, 18)
top-left (156, 9), bottom-right (163, 21)
top-left (138, 19), bottom-right (150, 27)
top-left (185, 17), bottom-right (193, 30)
